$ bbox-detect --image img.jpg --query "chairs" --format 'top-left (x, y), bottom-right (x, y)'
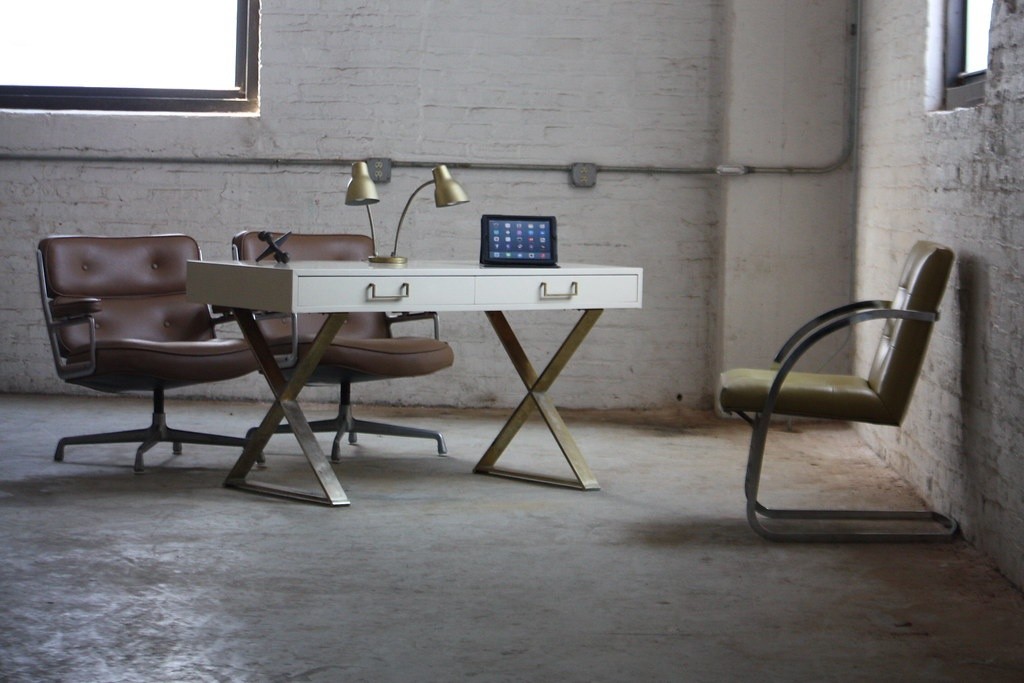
top-left (232, 230), bottom-right (454, 459)
top-left (34, 233), bottom-right (268, 476)
top-left (718, 239), bottom-right (961, 544)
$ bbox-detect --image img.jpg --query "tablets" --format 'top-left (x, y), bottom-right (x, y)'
top-left (480, 214), bottom-right (557, 265)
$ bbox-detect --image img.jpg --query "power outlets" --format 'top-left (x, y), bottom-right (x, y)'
top-left (571, 162), bottom-right (597, 187)
top-left (366, 158), bottom-right (393, 184)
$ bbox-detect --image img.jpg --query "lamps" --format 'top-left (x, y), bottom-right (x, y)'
top-left (344, 162), bottom-right (471, 263)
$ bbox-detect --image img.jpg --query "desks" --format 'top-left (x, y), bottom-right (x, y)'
top-left (188, 258), bottom-right (643, 509)
